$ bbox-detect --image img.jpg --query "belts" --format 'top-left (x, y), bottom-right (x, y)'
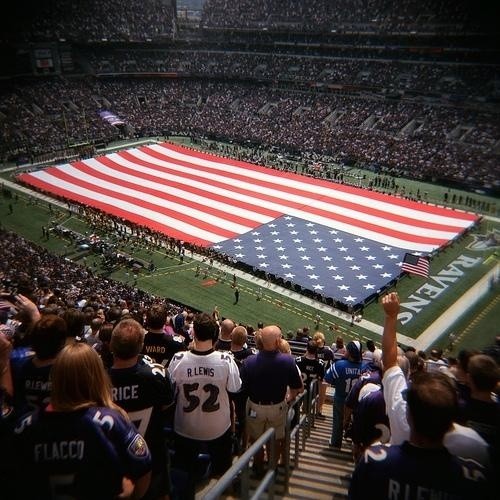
top-left (250, 399), bottom-right (282, 405)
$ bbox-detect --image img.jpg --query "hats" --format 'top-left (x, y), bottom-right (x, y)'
top-left (347, 341), bottom-right (362, 361)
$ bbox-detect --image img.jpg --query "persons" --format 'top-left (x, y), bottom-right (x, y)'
top-left (0, 0), bottom-right (500, 500)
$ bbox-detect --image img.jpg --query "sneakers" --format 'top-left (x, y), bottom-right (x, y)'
top-left (316, 413), bottom-right (325, 419)
